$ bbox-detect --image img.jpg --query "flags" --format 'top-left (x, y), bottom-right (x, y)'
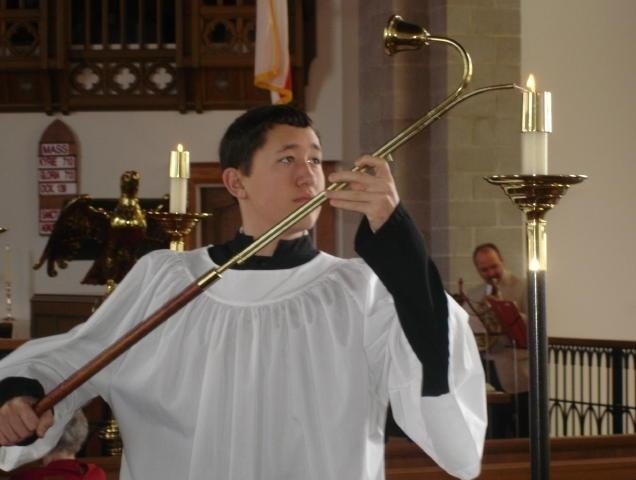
top-left (253, 0), bottom-right (293, 105)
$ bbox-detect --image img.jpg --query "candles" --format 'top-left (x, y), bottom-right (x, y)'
top-left (519, 73), bottom-right (553, 175)
top-left (168, 143), bottom-right (190, 214)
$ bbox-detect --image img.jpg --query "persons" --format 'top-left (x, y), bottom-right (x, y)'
top-left (460, 242), bottom-right (529, 440)
top-left (0, 105), bottom-right (490, 479)
